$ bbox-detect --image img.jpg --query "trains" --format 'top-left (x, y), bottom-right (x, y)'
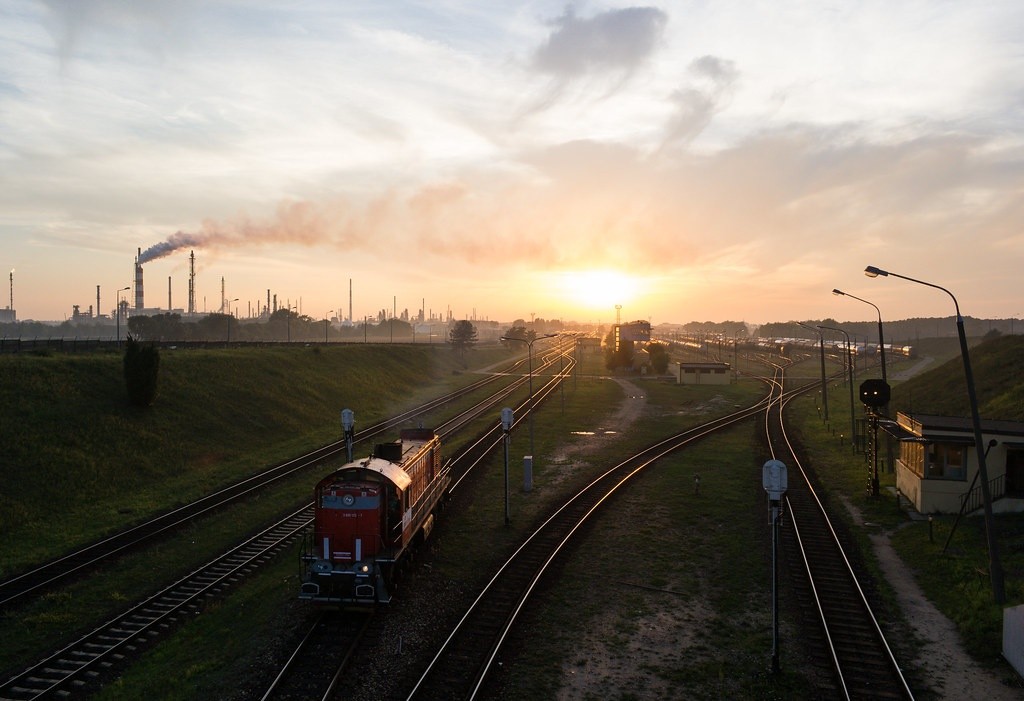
top-left (297, 423), bottom-right (455, 615)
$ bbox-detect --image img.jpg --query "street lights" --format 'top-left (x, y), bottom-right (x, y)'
top-left (326, 310), bottom-right (334, 341)
top-left (687, 327), bottom-right (727, 362)
top-left (795, 321), bottom-right (830, 433)
top-left (816, 324), bottom-right (858, 454)
top-left (831, 288), bottom-right (888, 500)
top-left (502, 333), bottom-right (559, 448)
top-left (544, 334), bottom-right (577, 417)
top-left (287, 307), bottom-right (297, 342)
top-left (117, 287), bottom-right (131, 341)
top-left (865, 264), bottom-right (1007, 607)
top-left (228, 298), bottom-right (239, 316)
top-left (734, 329), bottom-right (745, 386)
top-left (365, 315), bottom-right (374, 343)
